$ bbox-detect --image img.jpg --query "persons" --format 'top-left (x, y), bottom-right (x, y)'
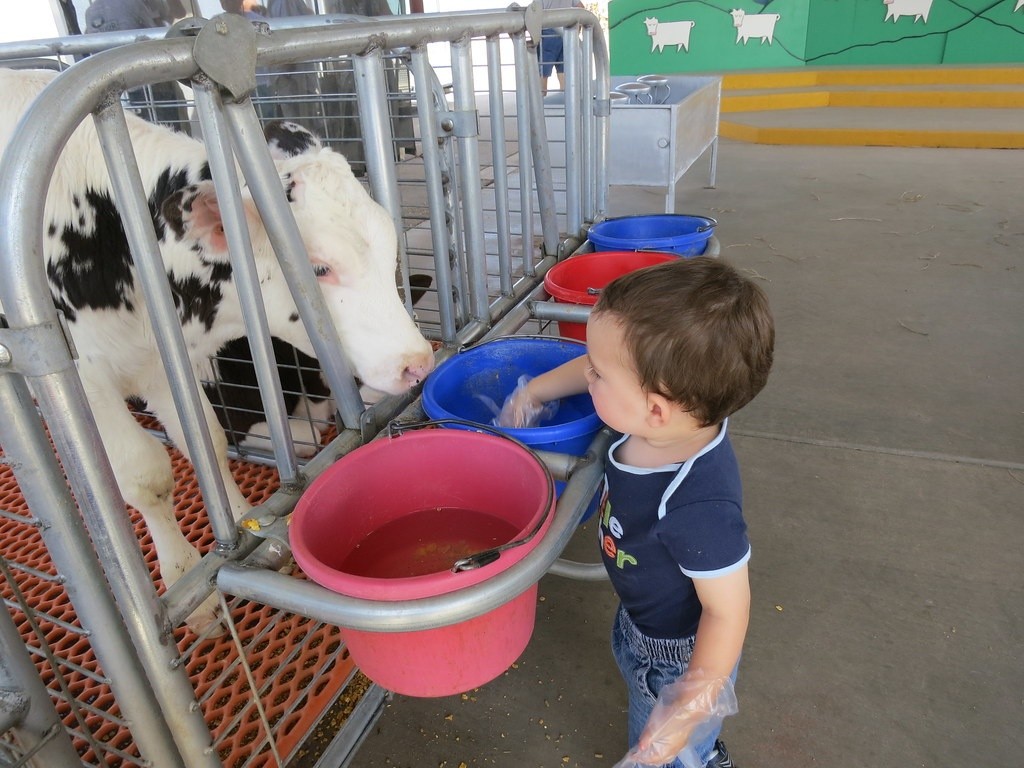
top-left (220, 0), bottom-right (275, 123)
top-left (533, 0), bottom-right (585, 96)
top-left (492, 257), bottom-right (774, 768)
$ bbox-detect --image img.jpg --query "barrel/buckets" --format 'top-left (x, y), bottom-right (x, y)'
top-left (590, 215), bottom-right (718, 256)
top-left (543, 252), bottom-right (682, 339)
top-left (421, 335), bottom-right (607, 523)
top-left (287, 422), bottom-right (555, 698)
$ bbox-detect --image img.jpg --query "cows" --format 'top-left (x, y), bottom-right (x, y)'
top-left (1, 66), bottom-right (436, 642)
